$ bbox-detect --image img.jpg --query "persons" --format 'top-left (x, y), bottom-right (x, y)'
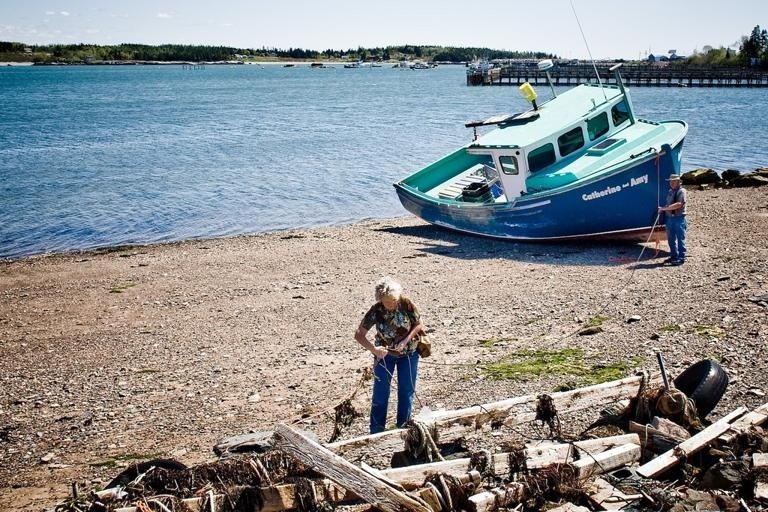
top-left (657, 174), bottom-right (688, 264)
top-left (354, 276), bottom-right (423, 433)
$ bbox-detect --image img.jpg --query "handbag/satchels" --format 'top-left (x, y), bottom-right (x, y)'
top-left (418, 329), bottom-right (431, 358)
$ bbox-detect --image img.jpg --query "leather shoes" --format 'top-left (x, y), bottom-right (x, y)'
top-left (665, 256), bottom-right (684, 265)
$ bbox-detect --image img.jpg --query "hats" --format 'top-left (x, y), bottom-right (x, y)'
top-left (665, 174), bottom-right (681, 181)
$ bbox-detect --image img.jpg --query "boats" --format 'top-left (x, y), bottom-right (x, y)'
top-left (392, 0), bottom-right (688, 244)
top-left (466, 60), bottom-right (494, 75)
top-left (282, 58), bottom-right (438, 70)
